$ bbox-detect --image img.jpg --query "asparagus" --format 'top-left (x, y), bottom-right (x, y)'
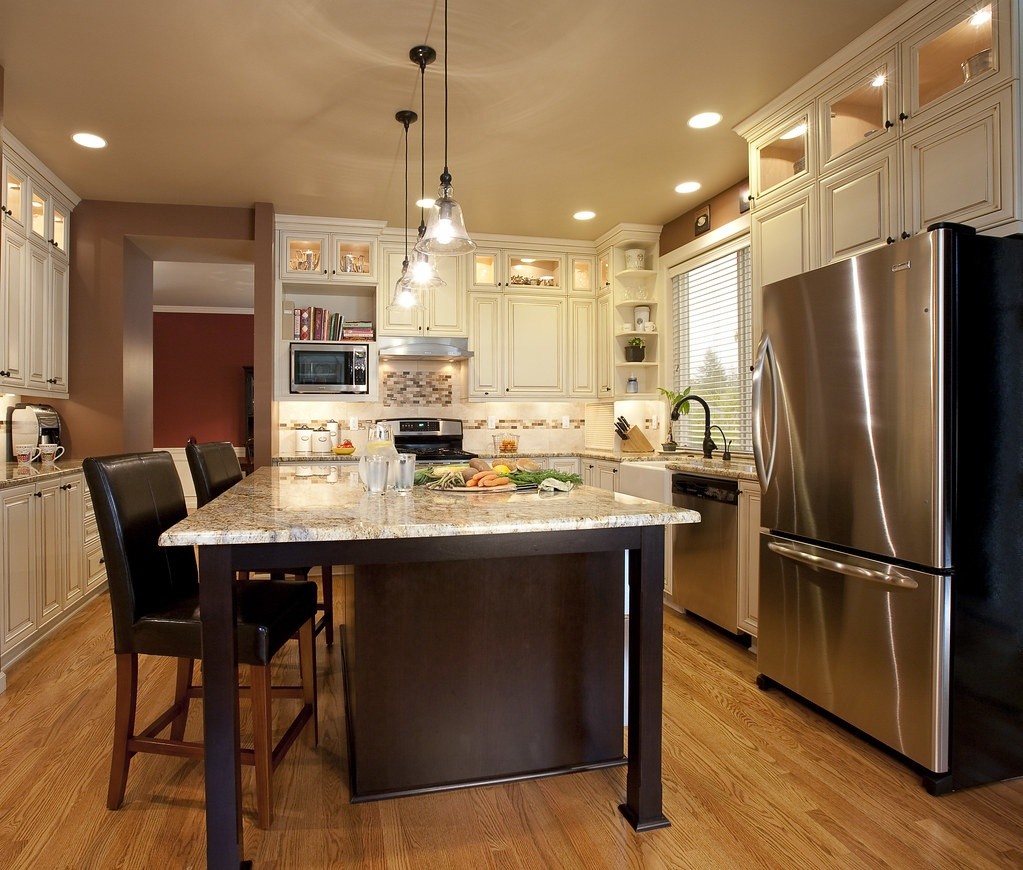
top-left (426, 472), bottom-right (466, 490)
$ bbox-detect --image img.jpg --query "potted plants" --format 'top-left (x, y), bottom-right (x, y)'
top-left (622, 338), bottom-right (646, 364)
top-left (659, 388), bottom-right (691, 452)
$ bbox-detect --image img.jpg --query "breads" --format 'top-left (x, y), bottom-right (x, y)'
top-left (493, 458), bottom-right (539, 471)
top-left (462, 458), bottom-right (492, 481)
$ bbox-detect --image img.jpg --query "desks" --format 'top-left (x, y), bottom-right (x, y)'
top-left (159, 463), bottom-right (702, 870)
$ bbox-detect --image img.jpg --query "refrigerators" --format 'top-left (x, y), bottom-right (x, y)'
top-left (748, 225), bottom-right (1023, 792)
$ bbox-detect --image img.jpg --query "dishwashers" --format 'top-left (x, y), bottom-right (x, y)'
top-left (670, 473), bottom-right (745, 636)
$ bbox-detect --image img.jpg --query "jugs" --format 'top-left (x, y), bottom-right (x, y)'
top-left (544, 279), bottom-right (558, 286)
top-left (511, 275), bottom-right (526, 285)
top-left (40, 431), bottom-right (56, 445)
top-left (526, 276), bottom-right (543, 286)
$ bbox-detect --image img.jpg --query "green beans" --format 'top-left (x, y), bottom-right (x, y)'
top-left (414, 467), bottom-right (436, 485)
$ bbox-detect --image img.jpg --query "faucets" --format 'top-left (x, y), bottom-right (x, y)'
top-left (671, 395), bottom-right (718, 460)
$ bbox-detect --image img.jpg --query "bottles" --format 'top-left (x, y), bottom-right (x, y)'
top-left (327, 419), bottom-right (339, 450)
top-left (295, 466), bottom-right (312, 477)
top-left (312, 427), bottom-right (331, 453)
top-left (624, 248), bottom-right (646, 270)
top-left (312, 466), bottom-right (330, 476)
top-left (626, 377), bottom-right (638, 393)
top-left (359, 424), bottom-right (399, 484)
top-left (326, 466), bottom-right (338, 485)
top-left (295, 425), bottom-right (314, 452)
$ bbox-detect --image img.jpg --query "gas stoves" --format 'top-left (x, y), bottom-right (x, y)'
top-left (376, 417), bottom-right (478, 460)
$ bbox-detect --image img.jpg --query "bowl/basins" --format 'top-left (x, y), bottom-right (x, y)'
top-left (492, 435), bottom-right (520, 452)
top-left (332, 447), bottom-right (356, 454)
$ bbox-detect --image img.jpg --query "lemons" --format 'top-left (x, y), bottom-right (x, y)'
top-left (493, 465), bottom-right (511, 474)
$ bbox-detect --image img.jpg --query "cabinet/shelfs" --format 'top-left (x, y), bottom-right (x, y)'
top-left (0, 472), bottom-right (108, 681)
top-left (268, 212), bottom-right (664, 453)
top-left (0, 125), bottom-right (81, 399)
top-left (734, 0), bottom-right (1023, 361)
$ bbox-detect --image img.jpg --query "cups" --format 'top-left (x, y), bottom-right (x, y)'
top-left (392, 453), bottom-right (416, 495)
top-left (364, 455), bottom-right (389, 495)
top-left (621, 323), bottom-right (631, 331)
top-left (16, 444), bottom-right (41, 464)
top-left (644, 321), bottom-right (655, 331)
top-left (634, 306), bottom-right (650, 331)
top-left (39, 444), bottom-right (65, 462)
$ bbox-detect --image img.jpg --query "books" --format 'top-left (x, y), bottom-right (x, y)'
top-left (282, 301), bottom-right (375, 341)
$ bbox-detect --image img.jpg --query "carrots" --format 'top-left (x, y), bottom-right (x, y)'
top-left (466, 471), bottom-right (510, 486)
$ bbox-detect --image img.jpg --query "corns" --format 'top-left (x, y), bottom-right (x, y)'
top-left (431, 467), bottom-right (466, 475)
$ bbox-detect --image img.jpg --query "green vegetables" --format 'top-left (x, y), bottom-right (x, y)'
top-left (500, 469), bottom-right (585, 486)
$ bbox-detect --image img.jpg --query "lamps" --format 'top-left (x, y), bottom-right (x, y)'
top-left (392, 110), bottom-right (424, 310)
top-left (416, 2), bottom-right (478, 258)
top-left (403, 45), bottom-right (446, 292)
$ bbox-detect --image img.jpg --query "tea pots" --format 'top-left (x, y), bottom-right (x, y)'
top-left (296, 249), bottom-right (320, 271)
top-left (340, 253), bottom-right (366, 273)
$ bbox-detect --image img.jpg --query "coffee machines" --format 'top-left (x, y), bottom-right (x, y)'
top-left (11, 403), bottom-right (62, 461)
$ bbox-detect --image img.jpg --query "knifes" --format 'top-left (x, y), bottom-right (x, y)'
top-left (614, 416), bottom-right (630, 440)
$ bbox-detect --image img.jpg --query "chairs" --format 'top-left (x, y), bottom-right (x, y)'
top-left (84, 449), bottom-right (322, 828)
top-left (184, 439), bottom-right (333, 673)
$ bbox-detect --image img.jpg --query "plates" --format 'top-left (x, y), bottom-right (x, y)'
top-left (434, 483), bottom-right (514, 491)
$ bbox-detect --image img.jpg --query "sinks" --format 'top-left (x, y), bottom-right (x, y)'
top-left (625, 461), bottom-right (674, 470)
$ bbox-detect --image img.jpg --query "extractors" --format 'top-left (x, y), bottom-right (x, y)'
top-left (378, 337), bottom-right (474, 362)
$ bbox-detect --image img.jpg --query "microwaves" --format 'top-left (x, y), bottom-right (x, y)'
top-left (291, 344), bottom-right (367, 393)
